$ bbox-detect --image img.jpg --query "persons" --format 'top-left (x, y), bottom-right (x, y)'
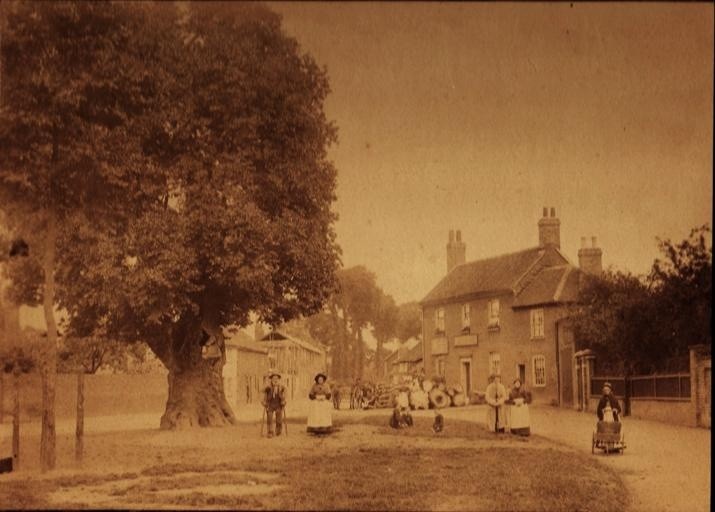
top-left (330, 365), bottom-right (446, 434)
top-left (486, 373), bottom-right (507, 432)
top-left (307, 373), bottom-right (334, 434)
top-left (262, 372), bottom-right (287, 439)
top-left (597, 380), bottom-right (621, 421)
top-left (510, 378), bottom-right (531, 435)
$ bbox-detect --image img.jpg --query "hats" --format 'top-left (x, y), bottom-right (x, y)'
top-left (268, 372), bottom-right (281, 379)
top-left (315, 373), bottom-right (327, 383)
